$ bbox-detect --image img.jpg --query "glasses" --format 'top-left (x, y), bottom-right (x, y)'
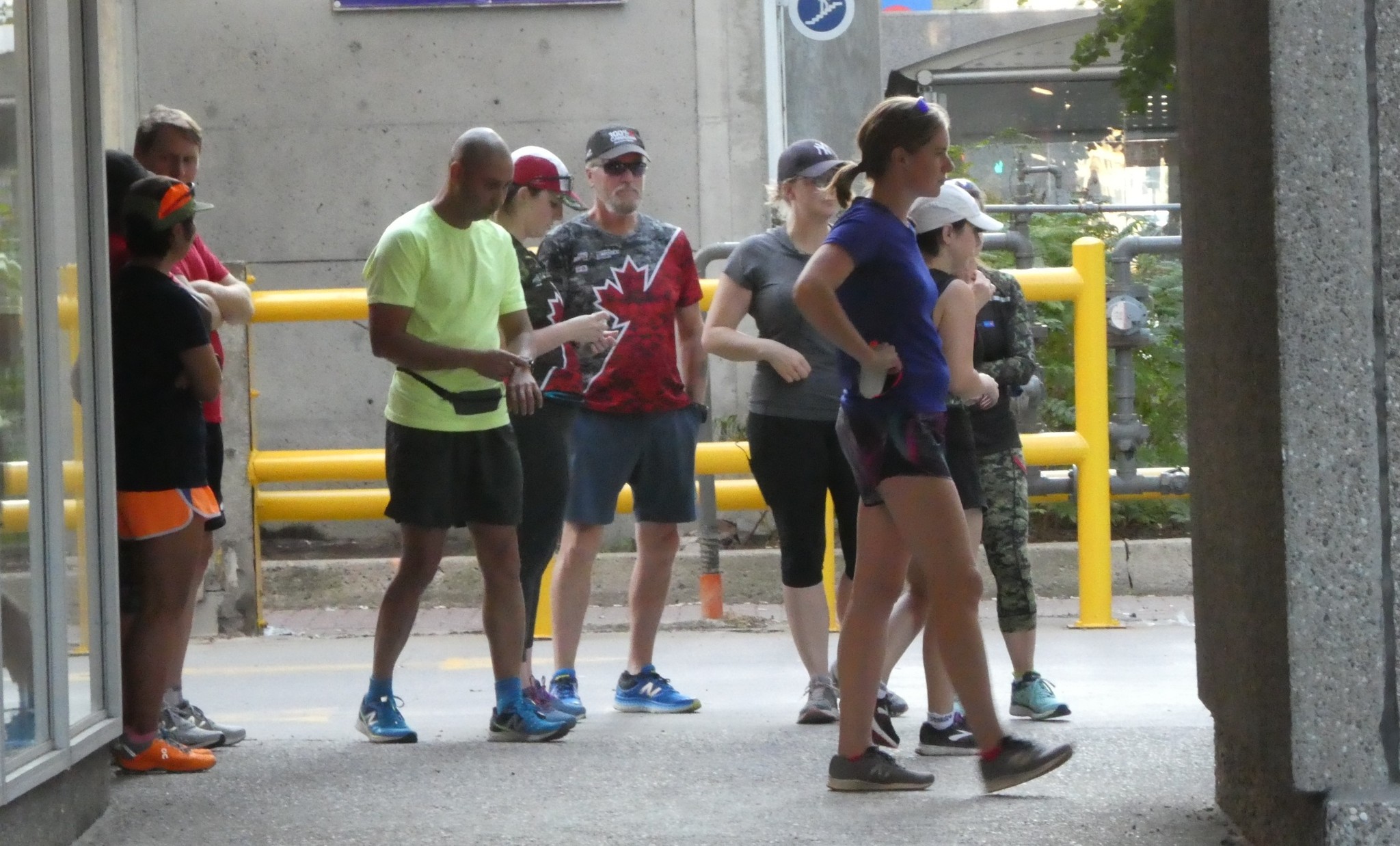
top-left (528, 174), bottom-right (575, 193)
top-left (175, 180), bottom-right (195, 203)
top-left (812, 175), bottom-right (831, 188)
top-left (913, 95), bottom-right (930, 115)
top-left (600, 160), bottom-right (647, 177)
top-left (957, 179), bottom-right (972, 190)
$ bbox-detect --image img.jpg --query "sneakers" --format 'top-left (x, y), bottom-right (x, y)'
top-left (354, 696), bottom-right (416, 743)
top-left (828, 747), bottom-right (935, 794)
top-left (1007, 670), bottom-right (1071, 721)
top-left (177, 699), bottom-right (246, 745)
top-left (882, 684), bottom-right (906, 716)
top-left (531, 677), bottom-right (585, 715)
top-left (155, 709), bottom-right (224, 748)
top-left (798, 664), bottom-right (841, 725)
top-left (914, 714), bottom-right (983, 755)
top-left (487, 706), bottom-right (568, 742)
top-left (979, 736), bottom-right (1072, 791)
top-left (871, 693), bottom-right (901, 748)
top-left (617, 665), bottom-right (700, 714)
top-left (523, 688), bottom-right (576, 728)
top-left (549, 668), bottom-right (585, 718)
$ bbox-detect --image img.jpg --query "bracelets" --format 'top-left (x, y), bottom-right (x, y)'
top-left (689, 400), bottom-right (710, 423)
top-left (514, 353), bottom-right (535, 374)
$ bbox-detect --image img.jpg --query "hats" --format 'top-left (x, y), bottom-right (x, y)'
top-left (907, 182), bottom-right (1003, 234)
top-left (105, 147), bottom-right (154, 224)
top-left (122, 173), bottom-right (214, 234)
top-left (777, 138), bottom-right (847, 179)
top-left (586, 125), bottom-right (651, 165)
top-left (511, 145), bottom-right (589, 212)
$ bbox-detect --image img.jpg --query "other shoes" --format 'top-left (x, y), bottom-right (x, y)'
top-left (116, 737), bottom-right (216, 770)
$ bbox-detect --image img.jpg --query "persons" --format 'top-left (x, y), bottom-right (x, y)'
top-left (99, 99), bottom-right (254, 753)
top-left (824, 177), bottom-right (1071, 757)
top-left (1, 587), bottom-right (35, 742)
top-left (703, 140), bottom-right (919, 725)
top-left (789, 95), bottom-right (1076, 798)
top-left (487, 147), bottom-right (621, 726)
top-left (356, 125), bottom-right (579, 744)
top-left (108, 173), bottom-right (223, 773)
top-left (530, 123), bottom-right (708, 721)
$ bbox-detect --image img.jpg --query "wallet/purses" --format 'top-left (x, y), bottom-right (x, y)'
top-left (397, 367), bottom-right (504, 416)
